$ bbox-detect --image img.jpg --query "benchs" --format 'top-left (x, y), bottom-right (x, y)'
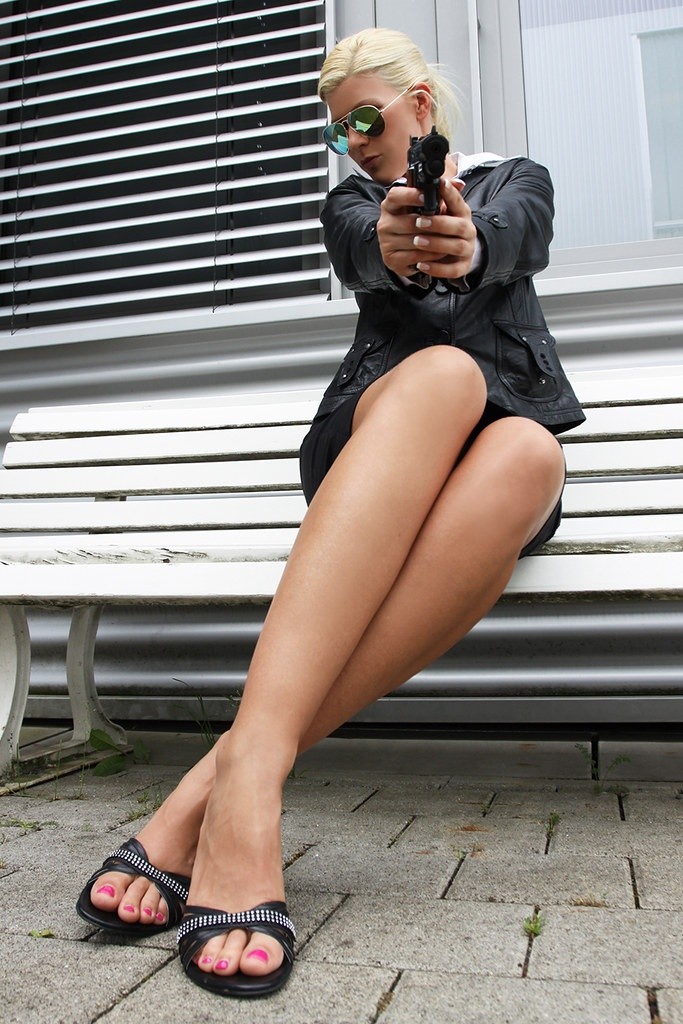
top-left (0, 364), bottom-right (683, 791)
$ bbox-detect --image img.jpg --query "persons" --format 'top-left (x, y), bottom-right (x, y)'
top-left (73, 26), bottom-right (587, 999)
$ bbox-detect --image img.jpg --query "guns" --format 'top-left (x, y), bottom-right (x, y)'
top-left (405, 124), bottom-right (451, 212)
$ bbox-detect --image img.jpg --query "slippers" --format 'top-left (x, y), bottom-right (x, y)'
top-left (177, 900), bottom-right (295, 997)
top-left (76, 838), bottom-right (191, 934)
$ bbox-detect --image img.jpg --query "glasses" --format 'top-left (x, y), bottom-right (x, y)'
top-left (322, 77), bottom-right (420, 156)
